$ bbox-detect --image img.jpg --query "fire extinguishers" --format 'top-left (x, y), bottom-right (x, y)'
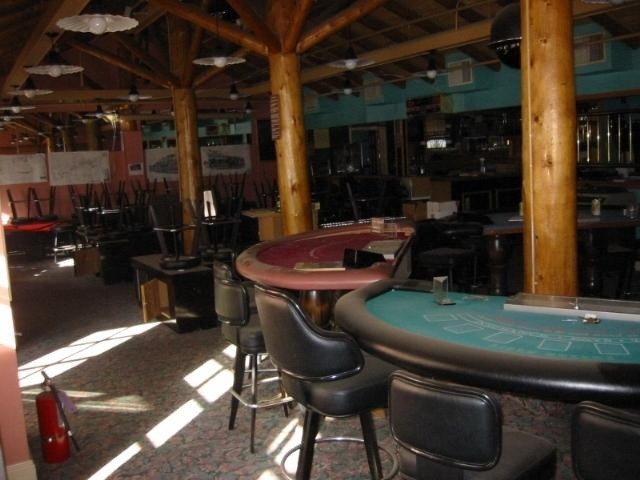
top-left (36, 370), bottom-right (80, 464)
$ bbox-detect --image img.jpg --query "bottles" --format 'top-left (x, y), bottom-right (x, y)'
top-left (591, 196), bottom-right (601, 216)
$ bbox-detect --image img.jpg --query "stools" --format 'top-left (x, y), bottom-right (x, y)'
top-left (389, 373), bottom-right (560, 477)
top-left (204, 258), bottom-right (303, 452)
top-left (572, 398), bottom-right (638, 480)
top-left (8, 170), bottom-right (638, 335)
top-left (248, 285), bottom-right (406, 479)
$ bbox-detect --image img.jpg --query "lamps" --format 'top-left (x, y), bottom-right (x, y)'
top-left (0, 66), bottom-right (152, 144)
top-left (331, 71), bottom-right (364, 96)
top-left (191, 0), bottom-right (247, 69)
top-left (55, 0), bottom-right (140, 35)
top-left (215, 68), bottom-right (251, 101)
top-left (24, 33), bottom-right (86, 78)
top-left (412, 49), bottom-right (454, 80)
top-left (324, 23), bottom-right (376, 71)
